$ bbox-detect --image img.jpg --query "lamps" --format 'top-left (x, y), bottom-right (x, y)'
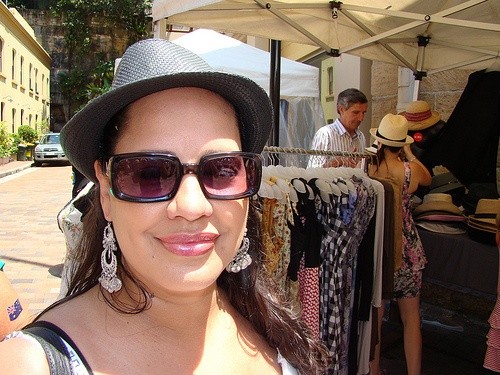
top-left (4, 95), bottom-right (13, 103)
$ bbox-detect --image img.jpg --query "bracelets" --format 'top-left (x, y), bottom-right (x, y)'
top-left (410, 155), bottom-right (416, 161)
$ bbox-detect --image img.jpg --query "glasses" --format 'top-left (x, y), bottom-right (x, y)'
top-left (98, 149), bottom-right (262, 203)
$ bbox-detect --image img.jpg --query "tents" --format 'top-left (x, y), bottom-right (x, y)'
top-left (151, 0), bottom-right (500, 165)
top-left (114, 28), bottom-right (326, 169)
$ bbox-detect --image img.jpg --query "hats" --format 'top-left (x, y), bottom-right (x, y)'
top-left (396, 100), bottom-right (441, 129)
top-left (369, 112), bottom-right (414, 146)
top-left (411, 165), bottom-right (500, 234)
top-left (60, 38), bottom-right (275, 185)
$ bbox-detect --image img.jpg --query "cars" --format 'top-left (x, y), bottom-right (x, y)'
top-left (33, 131), bottom-right (71, 167)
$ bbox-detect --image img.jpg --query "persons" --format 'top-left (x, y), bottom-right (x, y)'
top-left (366, 113), bottom-right (432, 375)
top-left (306, 89), bottom-right (367, 168)
top-left (0, 38), bottom-right (329, 375)
top-left (483, 199), bottom-right (500, 375)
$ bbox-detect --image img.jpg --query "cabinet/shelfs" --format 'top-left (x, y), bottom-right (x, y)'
top-left (417, 227), bottom-right (500, 367)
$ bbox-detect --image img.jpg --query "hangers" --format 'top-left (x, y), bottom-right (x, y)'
top-left (258, 146), bottom-right (393, 199)
